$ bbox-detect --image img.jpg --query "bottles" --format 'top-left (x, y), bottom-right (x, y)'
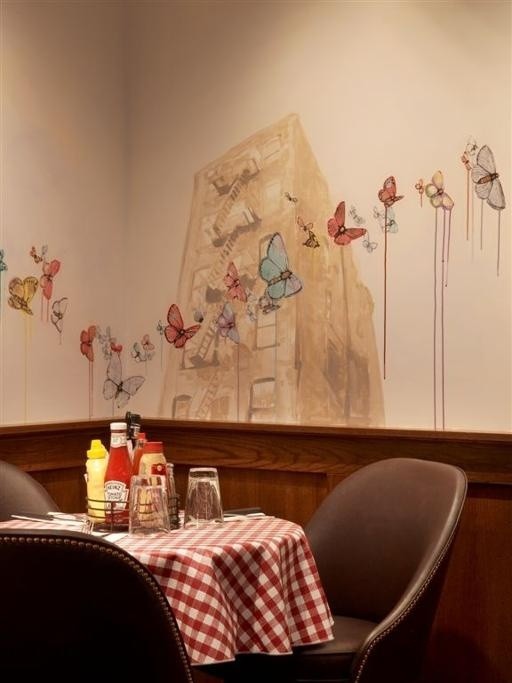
top-left (139, 440), bottom-right (169, 476)
top-left (129, 413), bottom-right (143, 446)
top-left (166, 463), bottom-right (177, 527)
top-left (133, 432), bottom-right (148, 474)
top-left (104, 420), bottom-right (133, 528)
top-left (86, 438), bottom-right (110, 522)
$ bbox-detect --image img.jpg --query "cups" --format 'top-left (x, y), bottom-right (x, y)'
top-left (183, 466), bottom-right (225, 531)
top-left (127, 474), bottom-right (171, 536)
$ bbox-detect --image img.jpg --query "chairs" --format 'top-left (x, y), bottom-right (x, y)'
top-left (241, 455), bottom-right (472, 681)
top-left (0, 457), bottom-right (57, 523)
top-left (0, 525), bottom-right (195, 683)
